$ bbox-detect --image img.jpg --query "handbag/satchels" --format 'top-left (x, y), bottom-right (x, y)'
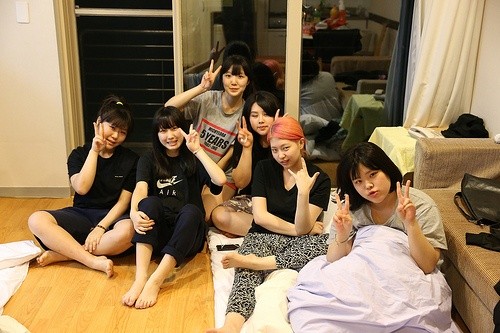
top-left (453, 172), bottom-right (500, 224)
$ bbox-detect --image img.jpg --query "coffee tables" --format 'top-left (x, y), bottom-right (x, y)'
top-left (369, 126), bottom-right (448, 190)
top-left (340, 94), bottom-right (388, 152)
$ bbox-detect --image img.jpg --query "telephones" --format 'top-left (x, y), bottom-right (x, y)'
top-left (374, 90), bottom-right (385, 101)
top-left (408, 126), bottom-right (445, 140)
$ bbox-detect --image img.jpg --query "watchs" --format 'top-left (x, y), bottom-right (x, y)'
top-left (192, 147), bottom-right (204, 157)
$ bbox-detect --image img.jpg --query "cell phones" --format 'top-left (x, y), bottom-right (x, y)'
top-left (216, 244), bottom-right (240, 251)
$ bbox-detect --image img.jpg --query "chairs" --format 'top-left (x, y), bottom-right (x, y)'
top-left (314, 28), bottom-right (361, 72)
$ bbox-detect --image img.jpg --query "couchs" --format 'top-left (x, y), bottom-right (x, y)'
top-left (413, 137), bottom-right (500, 333)
top-left (357, 80), bottom-right (387, 93)
top-left (330, 56), bottom-right (390, 86)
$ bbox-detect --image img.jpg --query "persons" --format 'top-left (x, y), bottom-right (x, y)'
top-left (261, 60), bottom-right (285, 86)
top-left (246, 62), bottom-right (276, 95)
top-left (326, 142), bottom-right (449, 274)
top-left (208, 116), bottom-right (332, 333)
top-left (27, 94), bottom-right (140, 278)
top-left (212, 89), bottom-right (289, 238)
top-left (180, 39), bottom-right (252, 93)
top-left (164, 59), bottom-right (250, 170)
top-left (121, 106), bottom-right (228, 309)
top-left (300, 50), bottom-right (343, 134)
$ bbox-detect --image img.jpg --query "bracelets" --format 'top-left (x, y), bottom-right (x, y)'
top-left (91, 224), bottom-right (106, 232)
top-left (334, 233), bottom-right (351, 245)
